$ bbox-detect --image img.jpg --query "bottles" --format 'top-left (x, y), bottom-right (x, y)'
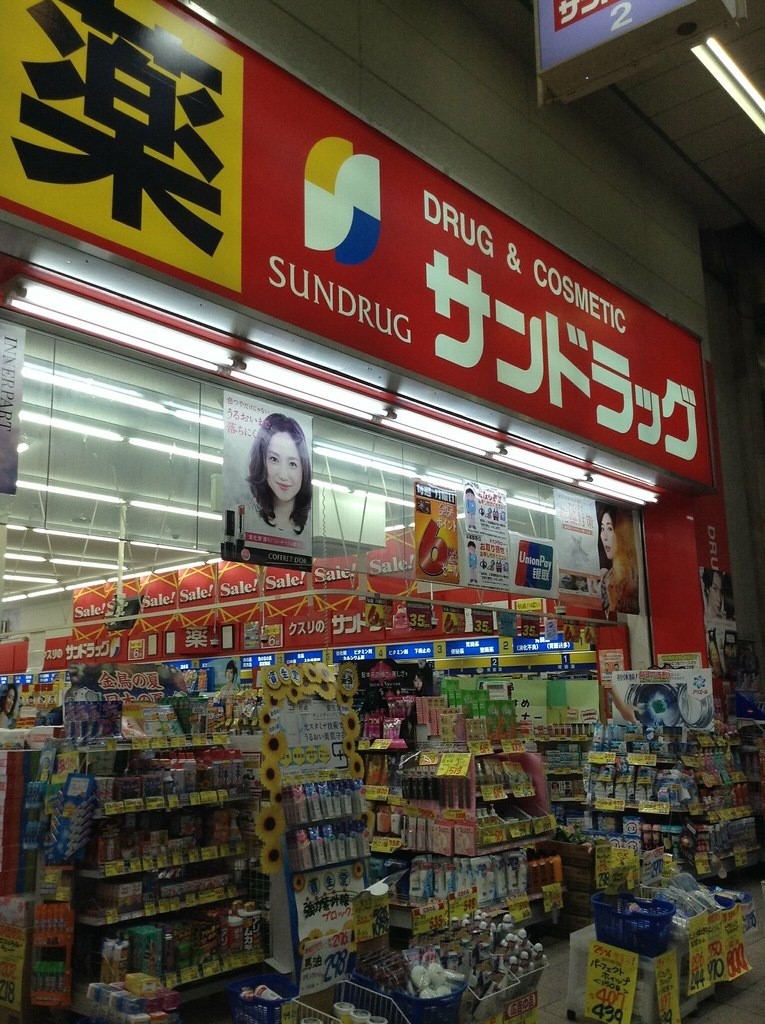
top-left (169, 752), bottom-right (197, 792)
top-left (163, 768), bottom-right (175, 799)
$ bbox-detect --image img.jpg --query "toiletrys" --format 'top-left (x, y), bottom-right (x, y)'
top-left (476, 804), bottom-right (500, 828)
top-left (433, 910), bottom-right (547, 1020)
top-left (408, 851), bottom-right (527, 903)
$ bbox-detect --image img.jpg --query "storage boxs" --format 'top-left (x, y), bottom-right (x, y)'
top-left (224, 973), bottom-right (297, 1024)
top-left (591, 889), bottom-right (677, 956)
top-left (352, 962), bottom-right (467, 1024)
top-left (124, 924), bottom-right (164, 995)
top-left (539, 743), bottom-right (643, 936)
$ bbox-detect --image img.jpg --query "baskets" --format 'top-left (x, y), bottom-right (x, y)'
top-left (354, 965), bottom-right (468, 1024)
top-left (591, 890), bottom-right (676, 958)
top-left (226, 973), bottom-right (298, 1024)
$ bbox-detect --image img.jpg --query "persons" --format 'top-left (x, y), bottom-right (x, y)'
top-left (597, 506), bottom-right (638, 614)
top-left (604, 680), bottom-right (640, 724)
top-left (228, 414), bottom-right (312, 543)
top-left (220, 660), bottom-right (237, 691)
top-left (0, 684), bottom-right (18, 728)
top-left (740, 670), bottom-right (759, 688)
top-left (702, 567), bottom-right (723, 619)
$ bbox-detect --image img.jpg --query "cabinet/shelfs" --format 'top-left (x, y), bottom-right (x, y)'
top-left (54, 730), bottom-right (271, 1005)
top-left (356, 726), bottom-right (563, 930)
top-left (535, 736), bottom-right (592, 839)
top-left (586, 738), bottom-right (765, 879)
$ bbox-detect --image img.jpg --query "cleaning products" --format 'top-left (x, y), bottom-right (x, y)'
top-left (99, 898), bottom-right (262, 983)
top-left (148, 748), bottom-right (243, 799)
